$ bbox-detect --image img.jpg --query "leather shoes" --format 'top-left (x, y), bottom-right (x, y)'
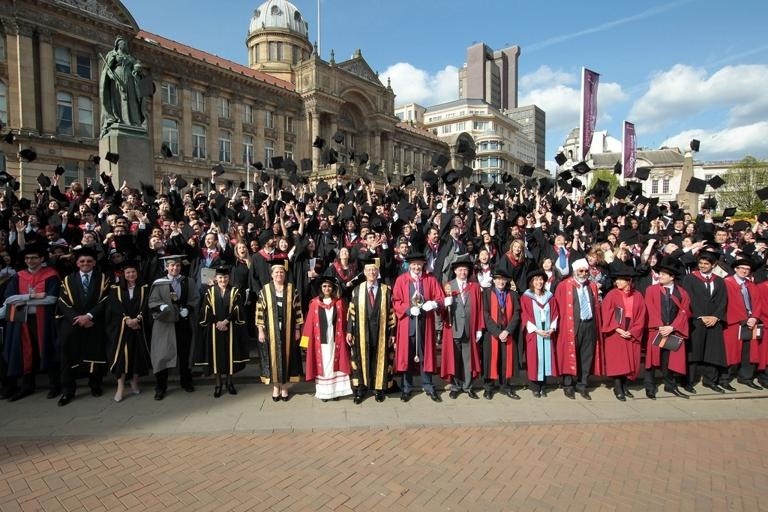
top-left (0, 391), bottom-right (35, 401)
top-left (89, 382), bottom-right (102, 396)
top-left (354, 374), bottom-right (768, 404)
top-left (47, 386), bottom-right (75, 406)
top-left (333, 398), bottom-right (339, 401)
top-left (181, 380), bottom-right (194, 392)
top-left (322, 399), bottom-right (327, 402)
top-left (155, 387), bottom-right (166, 399)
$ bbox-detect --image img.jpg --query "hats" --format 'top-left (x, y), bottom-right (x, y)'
top-left (0, 131), bottom-right (768, 287)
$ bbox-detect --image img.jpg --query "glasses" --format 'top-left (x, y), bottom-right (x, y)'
top-left (25, 257), bottom-right (40, 258)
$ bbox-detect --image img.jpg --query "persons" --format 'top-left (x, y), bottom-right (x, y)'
top-left (645, 266), bottom-right (690, 400)
top-left (520, 271), bottom-right (558, 398)
top-left (601, 272), bottom-right (646, 401)
top-left (753, 279), bottom-right (768, 390)
top-left (100, 37), bottom-right (149, 126)
top-left (683, 256), bottom-right (728, 392)
top-left (1, 169), bottom-right (259, 403)
top-left (554, 258), bottom-right (601, 399)
top-left (521, 177), bottom-right (767, 286)
top-left (723, 259), bottom-right (760, 390)
top-left (259, 168), bottom-right (520, 403)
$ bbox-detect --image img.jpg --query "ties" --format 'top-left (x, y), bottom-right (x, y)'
top-left (415, 275), bottom-right (419, 292)
top-left (460, 281), bottom-right (465, 303)
top-left (500, 292), bottom-right (505, 307)
top-left (579, 283), bottom-right (588, 322)
top-left (559, 246), bottom-right (568, 269)
top-left (83, 273), bottom-right (89, 293)
top-left (172, 278), bottom-right (179, 299)
top-left (706, 276), bottom-right (710, 296)
top-left (742, 282), bottom-right (750, 311)
top-left (666, 287), bottom-right (671, 323)
top-left (368, 286), bottom-right (374, 307)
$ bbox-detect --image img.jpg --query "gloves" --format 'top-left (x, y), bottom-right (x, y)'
top-left (476, 331), bottom-right (482, 342)
top-left (411, 307), bottom-right (419, 316)
top-left (160, 304), bottom-right (168, 311)
top-left (444, 297), bottom-right (453, 306)
top-left (422, 301), bottom-right (438, 311)
top-left (179, 308), bottom-right (188, 317)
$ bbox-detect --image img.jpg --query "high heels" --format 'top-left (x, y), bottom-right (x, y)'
top-left (281, 387), bottom-right (289, 402)
top-left (114, 392), bottom-right (123, 402)
top-left (215, 385), bottom-right (222, 397)
top-left (273, 386), bottom-right (280, 401)
top-left (226, 381), bottom-right (236, 394)
top-left (132, 389), bottom-right (141, 394)
